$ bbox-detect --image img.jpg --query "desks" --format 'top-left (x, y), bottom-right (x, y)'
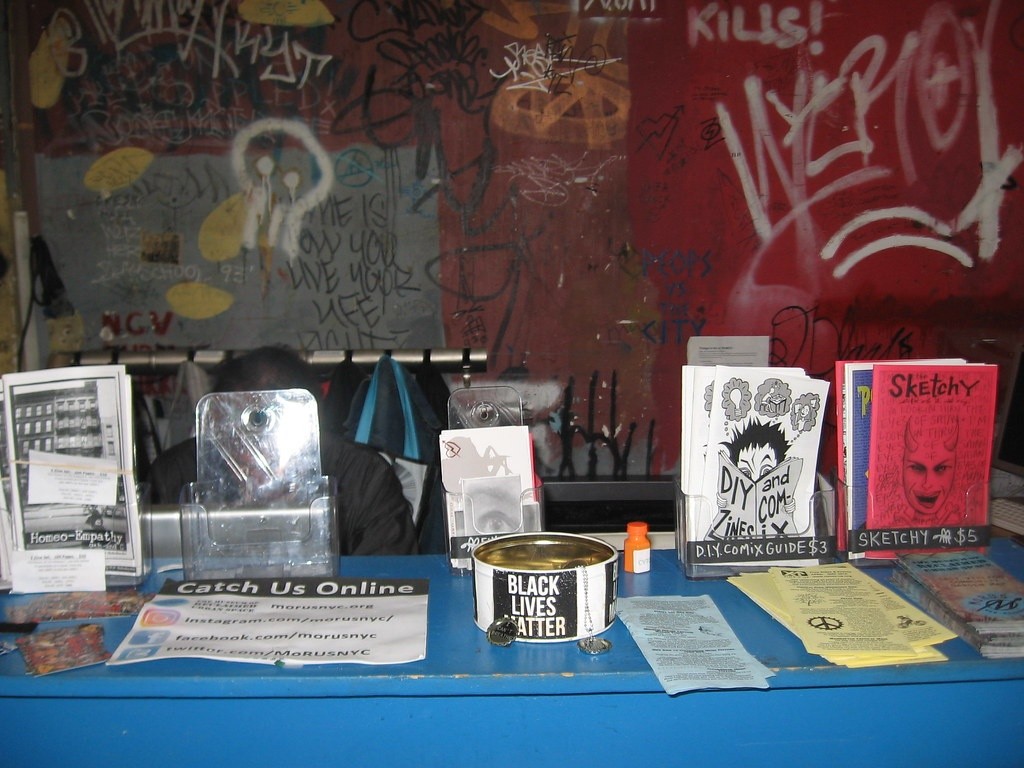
top-left (0, 539), bottom-right (1023, 768)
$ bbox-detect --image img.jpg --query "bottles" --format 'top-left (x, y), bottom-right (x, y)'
top-left (624, 521), bottom-right (652, 573)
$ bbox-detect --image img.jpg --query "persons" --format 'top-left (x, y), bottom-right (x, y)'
top-left (140, 344), bottom-right (421, 555)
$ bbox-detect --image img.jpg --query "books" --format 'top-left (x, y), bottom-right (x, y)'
top-left (835, 356), bottom-right (998, 567)
top-left (891, 551), bottom-right (1024, 660)
